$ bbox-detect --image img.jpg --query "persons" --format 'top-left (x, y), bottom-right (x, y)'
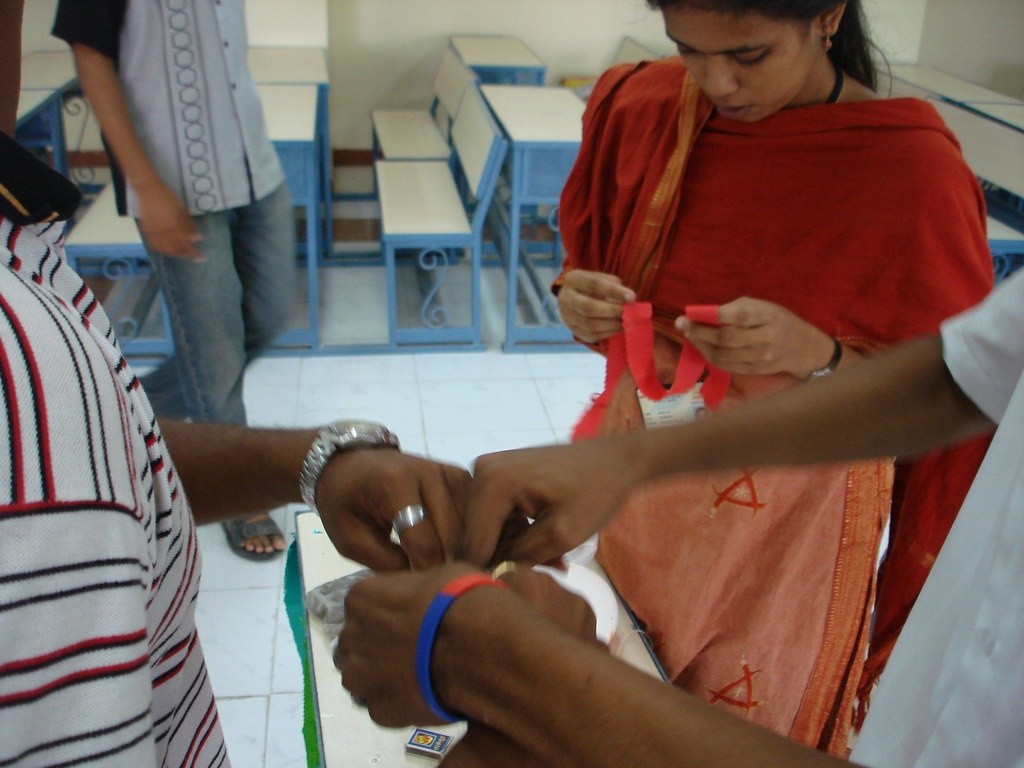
top-left (325, 259), bottom-right (1024, 768)
top-left (49, 0), bottom-right (296, 562)
top-left (549, 0), bottom-right (998, 734)
top-left (1, 0), bottom-right (610, 768)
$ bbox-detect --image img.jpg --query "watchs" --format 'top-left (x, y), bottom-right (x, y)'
top-left (809, 336), bottom-right (843, 382)
top-left (299, 420), bottom-right (401, 516)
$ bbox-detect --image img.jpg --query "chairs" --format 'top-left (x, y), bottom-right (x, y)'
top-left (365, 46), bottom-right (511, 355)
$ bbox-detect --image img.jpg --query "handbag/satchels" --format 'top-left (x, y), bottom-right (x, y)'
top-left (595, 302), bottom-right (892, 759)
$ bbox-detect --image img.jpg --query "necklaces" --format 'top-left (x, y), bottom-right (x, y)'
top-left (826, 64), bottom-right (843, 104)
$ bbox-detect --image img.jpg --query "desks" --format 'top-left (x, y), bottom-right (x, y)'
top-left (482, 82), bottom-right (602, 353)
top-left (252, 45), bottom-right (333, 259)
top-left (447, 31), bottom-right (548, 212)
top-left (21, 52), bottom-right (79, 149)
top-left (252, 83), bottom-right (328, 353)
top-left (17, 88), bottom-right (74, 237)
top-left (874, 63), bottom-right (1024, 208)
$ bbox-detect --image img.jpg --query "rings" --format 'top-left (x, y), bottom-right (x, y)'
top-left (490, 560), bottom-right (516, 580)
top-left (393, 504), bottom-right (428, 535)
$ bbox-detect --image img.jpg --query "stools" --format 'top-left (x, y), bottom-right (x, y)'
top-left (984, 218), bottom-right (1024, 286)
top-left (60, 187), bottom-right (176, 372)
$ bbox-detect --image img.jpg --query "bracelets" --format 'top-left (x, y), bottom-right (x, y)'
top-left (414, 574), bottom-right (508, 721)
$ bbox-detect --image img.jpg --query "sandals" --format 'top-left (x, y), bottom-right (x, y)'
top-left (222, 508), bottom-right (286, 562)
